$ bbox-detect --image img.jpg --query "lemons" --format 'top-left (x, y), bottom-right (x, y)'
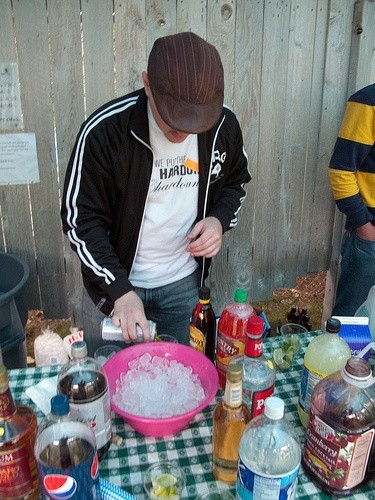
top-left (154, 483), bottom-right (175, 500)
top-left (273, 345), bottom-right (295, 370)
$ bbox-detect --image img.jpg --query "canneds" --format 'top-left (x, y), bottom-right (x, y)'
top-left (100, 316), bottom-right (157, 342)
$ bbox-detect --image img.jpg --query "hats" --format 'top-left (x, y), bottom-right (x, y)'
top-left (147, 31), bottom-right (225, 133)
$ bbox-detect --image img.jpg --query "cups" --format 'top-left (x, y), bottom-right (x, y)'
top-left (94, 345), bottom-right (122, 367)
top-left (143, 462), bottom-right (187, 500)
top-left (154, 335), bottom-right (178, 344)
top-left (193, 488), bottom-right (236, 500)
top-left (270, 323), bottom-right (308, 373)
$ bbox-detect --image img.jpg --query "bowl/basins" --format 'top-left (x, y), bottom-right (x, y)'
top-left (102, 343), bottom-right (219, 436)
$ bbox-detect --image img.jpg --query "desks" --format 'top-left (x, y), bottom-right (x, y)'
top-left (5, 330), bottom-right (375, 500)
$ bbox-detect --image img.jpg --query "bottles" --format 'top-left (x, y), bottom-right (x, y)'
top-left (0, 348), bottom-right (42, 500)
top-left (57, 341), bottom-right (112, 462)
top-left (212, 364), bottom-right (253, 485)
top-left (302, 342), bottom-right (375, 498)
top-left (229, 316), bottom-right (275, 426)
top-left (235, 396), bottom-right (302, 500)
top-left (34, 395), bottom-right (102, 500)
top-left (215, 288), bottom-right (257, 392)
top-left (297, 318), bottom-right (351, 430)
top-left (189, 287), bottom-right (216, 365)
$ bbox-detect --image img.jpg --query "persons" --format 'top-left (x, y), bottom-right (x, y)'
top-left (326, 82), bottom-right (375, 317)
top-left (59, 32), bottom-right (253, 346)
